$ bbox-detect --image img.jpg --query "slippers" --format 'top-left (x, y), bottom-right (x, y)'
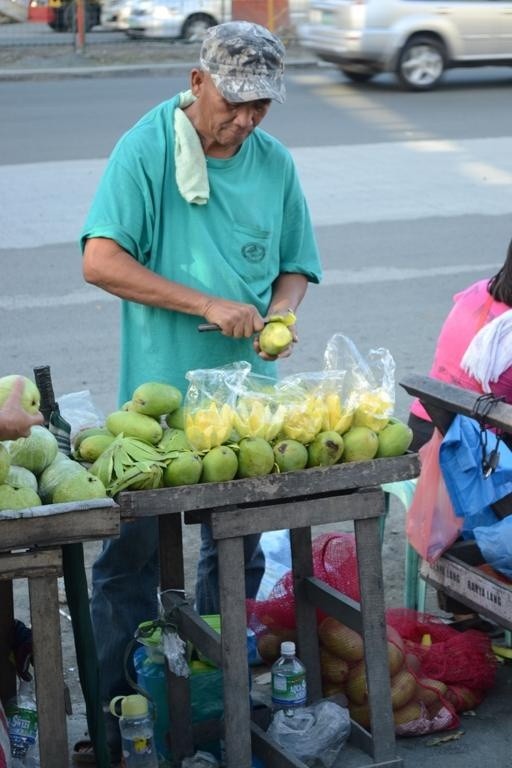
top-left (67, 724), bottom-right (125, 765)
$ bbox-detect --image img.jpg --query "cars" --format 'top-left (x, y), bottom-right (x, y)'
top-left (299, 1), bottom-right (512, 91)
top-left (100, 0), bottom-right (232, 42)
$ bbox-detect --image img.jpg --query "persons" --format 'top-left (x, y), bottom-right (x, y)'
top-left (78, 22), bottom-right (321, 768)
top-left (0, 377), bottom-right (46, 441)
top-left (404, 239), bottom-right (512, 638)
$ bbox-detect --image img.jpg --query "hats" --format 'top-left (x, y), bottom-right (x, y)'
top-left (197, 20), bottom-right (288, 107)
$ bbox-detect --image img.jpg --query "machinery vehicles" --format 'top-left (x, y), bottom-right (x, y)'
top-left (28, 0), bottom-right (99, 33)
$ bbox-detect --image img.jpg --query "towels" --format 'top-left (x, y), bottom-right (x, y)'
top-left (462, 309), bottom-right (512, 395)
top-left (174, 90), bottom-right (211, 205)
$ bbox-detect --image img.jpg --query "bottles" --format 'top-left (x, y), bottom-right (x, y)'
top-left (9, 677), bottom-right (38, 758)
top-left (270, 641), bottom-right (307, 716)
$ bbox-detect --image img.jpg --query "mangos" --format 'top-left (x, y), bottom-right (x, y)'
top-left (256, 614), bottom-right (484, 735)
top-left (259, 313), bottom-right (297, 359)
top-left (0, 374), bottom-right (107, 514)
top-left (74, 379), bottom-right (413, 490)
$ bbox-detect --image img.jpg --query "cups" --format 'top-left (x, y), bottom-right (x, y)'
top-left (110, 695), bottom-right (159, 768)
